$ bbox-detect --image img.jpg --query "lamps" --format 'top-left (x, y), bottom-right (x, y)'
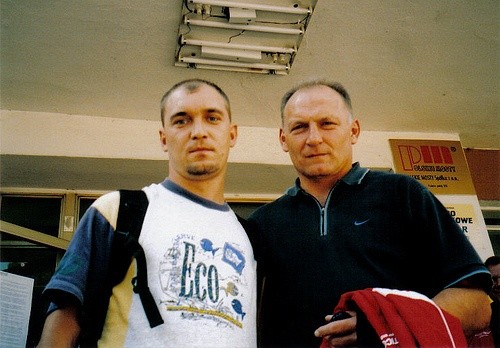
top-left (178, 0), bottom-right (312, 71)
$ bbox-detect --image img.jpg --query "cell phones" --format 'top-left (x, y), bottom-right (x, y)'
top-left (330, 311), bottom-right (354, 338)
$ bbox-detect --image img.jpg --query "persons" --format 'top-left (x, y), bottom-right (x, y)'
top-left (484, 256), bottom-right (500, 331)
top-left (36, 78), bottom-right (273, 348)
top-left (245, 79), bottom-right (496, 348)
